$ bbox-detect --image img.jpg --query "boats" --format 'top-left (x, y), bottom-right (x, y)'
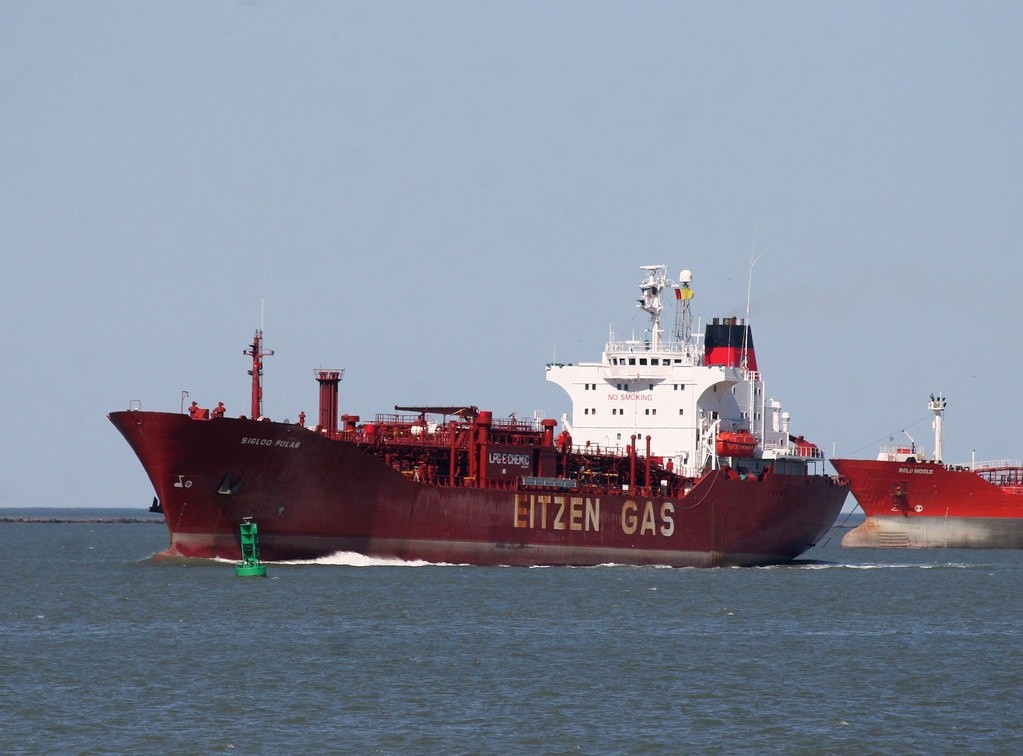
top-left (106, 220), bottom-right (851, 568)
top-left (715, 432), bottom-right (757, 457)
top-left (829, 391), bottom-right (1023, 549)
